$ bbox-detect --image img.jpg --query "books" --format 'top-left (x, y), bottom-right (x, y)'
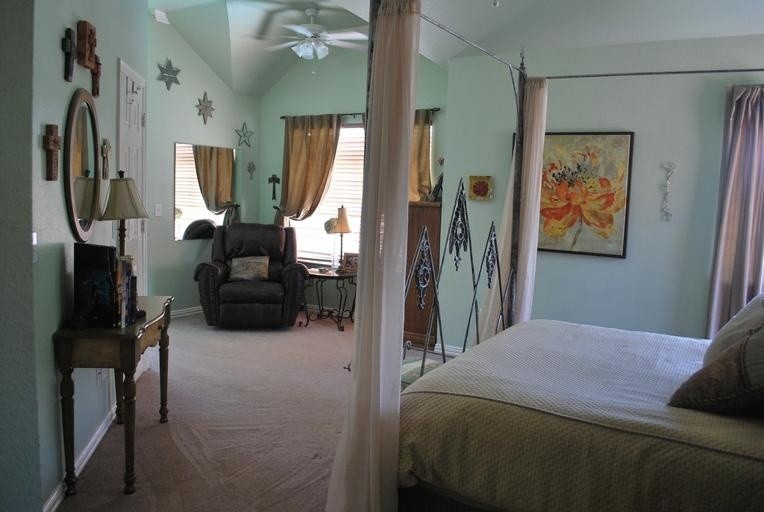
top-left (69, 239), bottom-right (138, 330)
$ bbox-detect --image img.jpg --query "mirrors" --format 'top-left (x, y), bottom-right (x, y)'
top-left (62, 88), bottom-right (102, 243)
top-left (172, 142), bottom-right (236, 242)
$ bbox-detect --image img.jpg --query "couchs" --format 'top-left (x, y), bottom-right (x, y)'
top-left (181, 219), bottom-right (215, 240)
top-left (193, 222), bottom-right (308, 330)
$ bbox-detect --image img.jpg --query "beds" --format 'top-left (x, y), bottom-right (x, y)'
top-left (323, 0), bottom-right (762, 510)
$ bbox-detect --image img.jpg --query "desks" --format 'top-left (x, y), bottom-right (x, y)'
top-left (49, 294), bottom-right (173, 494)
top-left (298, 268), bottom-right (358, 331)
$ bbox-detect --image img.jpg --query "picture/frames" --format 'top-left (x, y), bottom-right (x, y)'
top-left (342, 253), bottom-right (357, 272)
top-left (511, 131), bottom-right (635, 260)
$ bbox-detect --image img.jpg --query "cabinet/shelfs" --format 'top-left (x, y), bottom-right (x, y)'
top-left (402, 202), bottom-right (441, 351)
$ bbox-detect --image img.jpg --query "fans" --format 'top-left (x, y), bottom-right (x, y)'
top-left (255, 8), bottom-right (371, 63)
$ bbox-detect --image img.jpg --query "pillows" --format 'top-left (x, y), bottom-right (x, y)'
top-left (228, 256), bottom-right (270, 281)
top-left (667, 328), bottom-right (762, 417)
top-left (703, 288), bottom-right (760, 370)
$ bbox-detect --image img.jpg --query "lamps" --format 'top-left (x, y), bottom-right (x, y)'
top-left (333, 206), bottom-right (351, 273)
top-left (73, 170), bottom-right (94, 228)
top-left (98, 170), bottom-right (150, 320)
